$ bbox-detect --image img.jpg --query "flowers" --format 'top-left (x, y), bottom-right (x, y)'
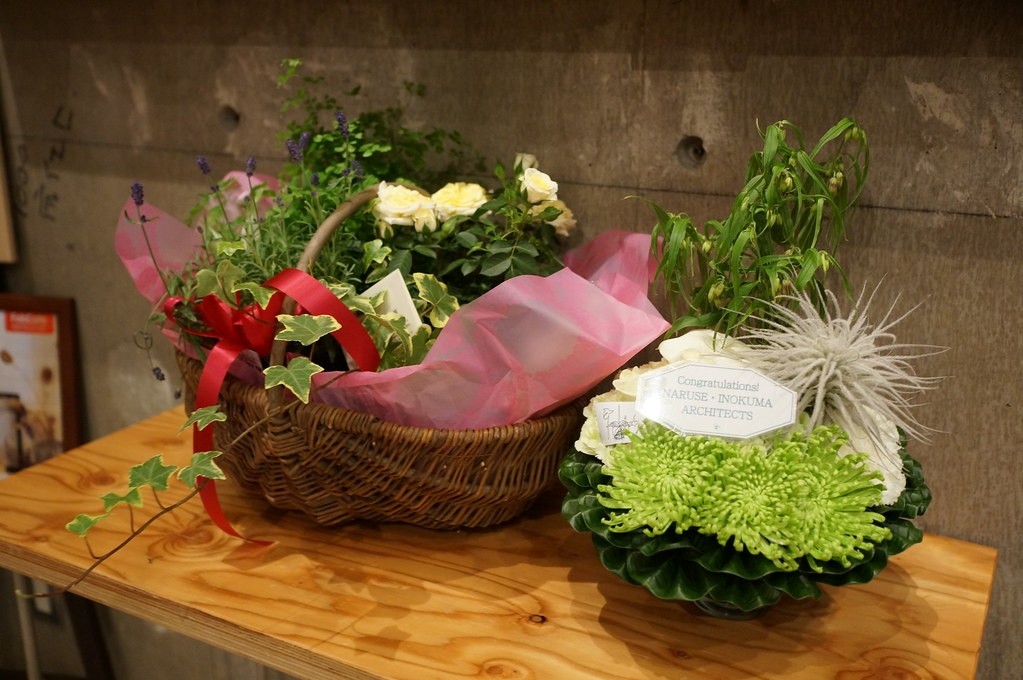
top-left (112, 57), bottom-right (573, 374)
top-left (562, 116), bottom-right (940, 617)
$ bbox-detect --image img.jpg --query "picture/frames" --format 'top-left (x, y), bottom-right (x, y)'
top-left (1, 292), bottom-right (88, 617)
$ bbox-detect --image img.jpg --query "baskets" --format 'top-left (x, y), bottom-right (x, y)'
top-left (176, 181), bottom-right (599, 530)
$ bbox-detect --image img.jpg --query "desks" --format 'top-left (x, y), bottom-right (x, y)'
top-left (1, 408), bottom-right (998, 680)
